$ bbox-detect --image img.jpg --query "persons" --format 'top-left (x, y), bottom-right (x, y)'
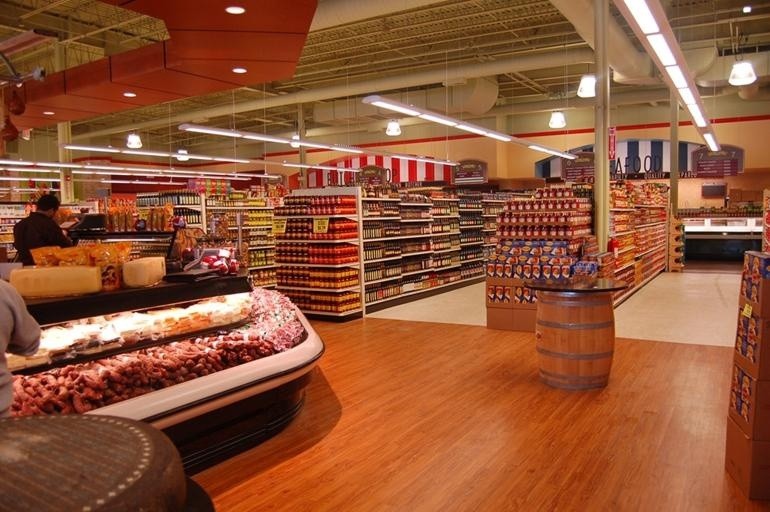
top-left (0, 277), bottom-right (42, 423)
top-left (13, 194), bottom-right (74, 267)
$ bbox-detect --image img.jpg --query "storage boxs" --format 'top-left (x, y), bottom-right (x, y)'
top-left (723, 250), bottom-right (769, 499)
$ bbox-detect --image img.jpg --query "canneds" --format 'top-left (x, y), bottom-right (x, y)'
top-left (274, 196), bottom-right (361, 312)
top-left (495, 188), bottom-right (592, 236)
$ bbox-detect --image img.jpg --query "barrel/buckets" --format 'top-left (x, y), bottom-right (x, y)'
top-left (535, 291), bottom-right (614, 389)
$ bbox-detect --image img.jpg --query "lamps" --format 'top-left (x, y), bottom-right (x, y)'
top-left (613, 0), bottom-right (757, 153)
top-left (1, 74), bottom-right (597, 185)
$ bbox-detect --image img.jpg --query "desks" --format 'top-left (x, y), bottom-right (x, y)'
top-left (2, 414), bottom-right (188, 510)
top-left (522, 276), bottom-right (630, 390)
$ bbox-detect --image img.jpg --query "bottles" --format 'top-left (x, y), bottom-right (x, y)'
top-left (135, 189), bottom-right (202, 225)
top-left (273, 194), bottom-right (361, 314)
top-left (361, 188), bottom-right (484, 304)
top-left (205, 192), bottom-right (277, 287)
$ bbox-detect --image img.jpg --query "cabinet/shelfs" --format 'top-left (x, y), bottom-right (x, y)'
top-left (1, 185), bottom-right (687, 334)
top-left (677, 186), bottom-right (769, 253)
top-left (2, 287), bottom-right (325, 476)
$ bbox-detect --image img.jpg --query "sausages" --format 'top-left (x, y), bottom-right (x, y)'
top-left (9, 333), bottom-right (272, 416)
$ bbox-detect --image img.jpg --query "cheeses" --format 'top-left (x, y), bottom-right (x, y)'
top-left (123, 257), bottom-right (166, 287)
top-left (10, 266), bottom-right (102, 298)
